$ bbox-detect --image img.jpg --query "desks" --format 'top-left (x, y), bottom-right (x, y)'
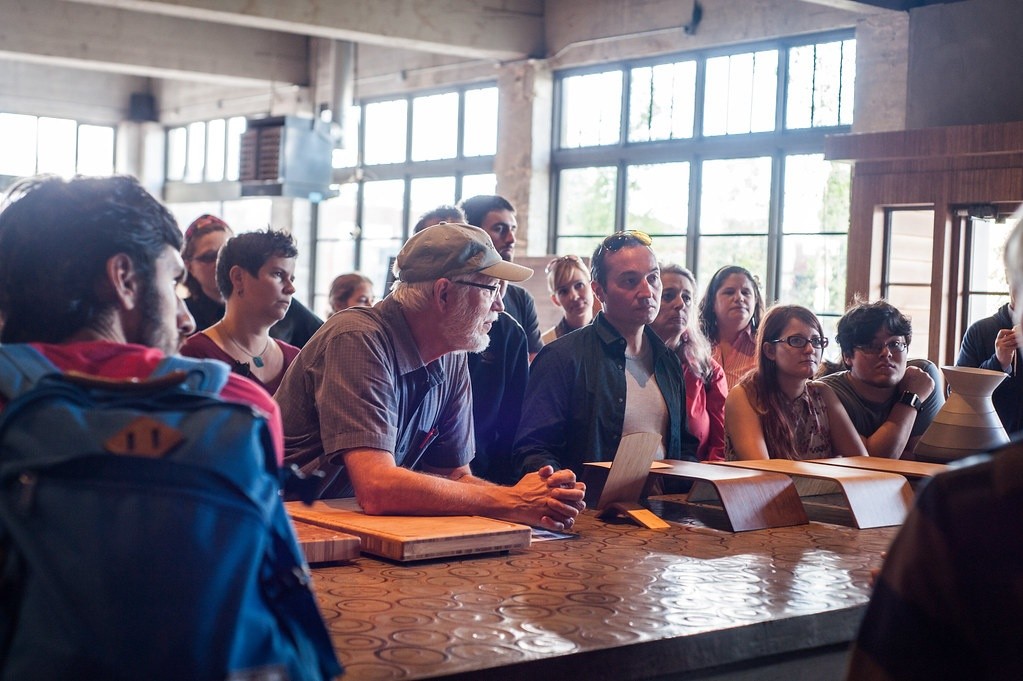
top-left (302, 491), bottom-right (905, 681)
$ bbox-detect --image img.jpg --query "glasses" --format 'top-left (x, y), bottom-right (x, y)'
top-left (187, 249), bottom-right (217, 263)
top-left (765, 335), bottom-right (829, 349)
top-left (854, 340), bottom-right (910, 355)
top-left (597, 230), bottom-right (653, 255)
top-left (452, 280), bottom-right (500, 298)
top-left (545, 255), bottom-right (578, 273)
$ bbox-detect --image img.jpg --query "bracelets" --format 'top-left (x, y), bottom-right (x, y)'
top-left (898, 390), bottom-right (923, 413)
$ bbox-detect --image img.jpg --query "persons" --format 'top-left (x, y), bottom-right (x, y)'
top-left (849, 219), bottom-right (1023, 681)
top-left (954, 247), bottom-right (1023, 442)
top-left (330, 274), bottom-right (373, 311)
top-left (271, 221), bottom-right (587, 533)
top-left (176, 228), bottom-right (303, 391)
top-left (540, 255), bottom-right (594, 344)
top-left (178, 215), bottom-right (325, 348)
top-left (818, 301), bottom-right (945, 459)
top-left (650, 265), bottom-right (729, 459)
top-left (699, 266), bottom-right (764, 390)
top-left (0, 175), bottom-right (286, 464)
top-left (725, 304), bottom-right (870, 462)
top-left (461, 194), bottom-right (544, 360)
top-left (511, 230), bottom-right (700, 508)
top-left (415, 207), bottom-right (529, 483)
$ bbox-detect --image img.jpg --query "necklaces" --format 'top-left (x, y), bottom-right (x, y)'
top-left (221, 320), bottom-right (269, 367)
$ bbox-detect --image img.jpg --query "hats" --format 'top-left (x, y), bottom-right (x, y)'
top-left (182, 215), bottom-right (230, 260)
top-left (396, 223), bottom-right (534, 281)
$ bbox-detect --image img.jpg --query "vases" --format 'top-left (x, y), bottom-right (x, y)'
top-left (913, 365), bottom-right (1008, 464)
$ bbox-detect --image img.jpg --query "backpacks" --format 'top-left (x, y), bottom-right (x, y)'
top-left (0, 342), bottom-right (342, 681)
